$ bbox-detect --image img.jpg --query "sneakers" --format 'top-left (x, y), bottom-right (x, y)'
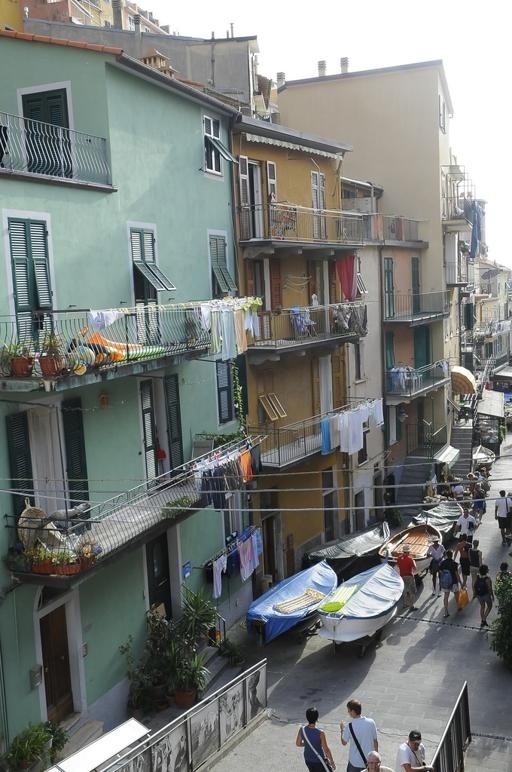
top-left (410, 606), bottom-right (419, 611)
top-left (481, 620), bottom-right (489, 628)
top-left (432, 590), bottom-right (442, 597)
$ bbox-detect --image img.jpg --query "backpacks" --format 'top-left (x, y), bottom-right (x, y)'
top-left (474, 579), bottom-right (488, 596)
top-left (439, 569), bottom-right (453, 590)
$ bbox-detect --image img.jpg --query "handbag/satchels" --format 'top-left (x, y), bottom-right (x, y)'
top-left (325, 757), bottom-right (334, 772)
top-left (459, 588), bottom-right (469, 609)
top-left (506, 512), bottom-right (512, 522)
top-left (467, 521), bottom-right (474, 530)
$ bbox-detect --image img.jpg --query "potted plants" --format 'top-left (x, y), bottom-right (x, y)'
top-left (38, 332), bottom-right (64, 380)
top-left (0, 719), bottom-right (70, 772)
top-left (118, 634), bottom-right (206, 706)
top-left (2, 340), bottom-right (34, 377)
top-left (46, 550), bottom-right (80, 575)
top-left (23, 544), bottom-right (54, 574)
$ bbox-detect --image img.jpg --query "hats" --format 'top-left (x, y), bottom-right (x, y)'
top-left (409, 731), bottom-right (422, 743)
top-left (429, 537), bottom-right (441, 542)
top-left (403, 545), bottom-right (411, 552)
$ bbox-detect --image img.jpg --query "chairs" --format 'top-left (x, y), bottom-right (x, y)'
top-left (403, 365), bottom-right (422, 391)
top-left (291, 305), bottom-right (319, 341)
top-left (192, 439), bottom-right (214, 460)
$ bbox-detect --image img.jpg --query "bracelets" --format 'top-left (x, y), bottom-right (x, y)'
top-left (340, 729), bottom-right (344, 732)
top-left (332, 765), bottom-right (335, 768)
top-left (330, 761), bottom-right (334, 763)
top-left (422, 766), bottom-right (427, 771)
top-left (330, 763), bottom-right (334, 765)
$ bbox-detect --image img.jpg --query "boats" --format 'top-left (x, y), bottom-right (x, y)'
top-left (247, 497), bottom-right (472, 650)
top-left (473, 444), bottom-right (495, 472)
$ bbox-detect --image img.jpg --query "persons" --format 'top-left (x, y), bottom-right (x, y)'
top-left (296, 708), bottom-right (336, 771)
top-left (340, 699), bottom-right (379, 771)
top-left (360, 751), bottom-right (395, 771)
top-left (396, 730), bottom-right (435, 771)
top-left (396, 467), bottom-right (512, 627)
